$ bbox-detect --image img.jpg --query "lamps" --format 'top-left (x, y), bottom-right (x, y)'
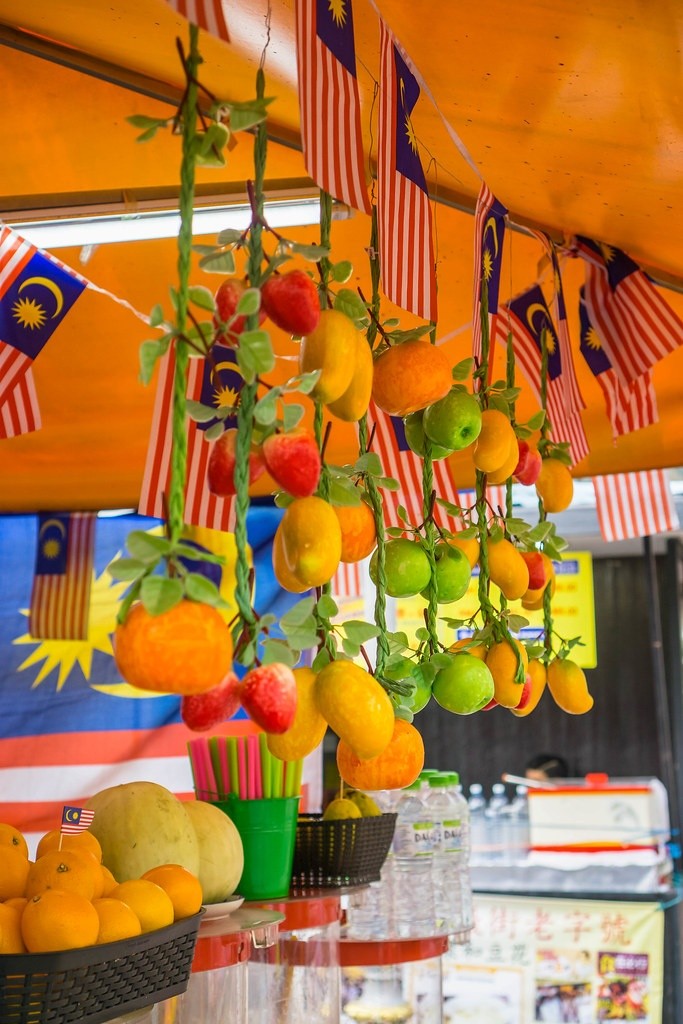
top-left (0, 176), bottom-right (356, 247)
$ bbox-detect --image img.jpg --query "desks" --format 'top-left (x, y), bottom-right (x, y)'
top-left (473, 884), bottom-right (683, 1024)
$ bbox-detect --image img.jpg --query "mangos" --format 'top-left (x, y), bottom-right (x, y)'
top-left (263, 312), bottom-right (397, 762)
top-left (446, 410), bottom-right (594, 717)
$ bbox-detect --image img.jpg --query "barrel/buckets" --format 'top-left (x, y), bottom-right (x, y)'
top-left (191, 785), bottom-right (302, 902)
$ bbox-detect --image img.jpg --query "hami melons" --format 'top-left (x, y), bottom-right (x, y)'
top-left (84, 781), bottom-right (198, 885)
top-left (183, 799), bottom-right (245, 905)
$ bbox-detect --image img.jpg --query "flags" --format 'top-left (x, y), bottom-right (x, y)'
top-left (455, 483), bottom-right (506, 545)
top-left (472, 181), bottom-right (507, 407)
top-left (27, 508), bottom-right (95, 640)
top-left (167, 0), bottom-right (229, 43)
top-left (377, 16), bottom-right (438, 324)
top-left (61, 806), bottom-right (95, 835)
top-left (293, 0), bottom-right (372, 214)
top-left (0, 226), bottom-right (86, 439)
top-left (322, 561), bottom-right (359, 598)
top-left (0, 499), bottom-right (324, 1024)
top-left (138, 334), bottom-right (254, 533)
top-left (533, 227), bottom-right (683, 438)
top-left (495, 286), bottom-right (589, 466)
top-left (594, 468), bottom-right (679, 542)
top-left (356, 393), bottom-right (463, 538)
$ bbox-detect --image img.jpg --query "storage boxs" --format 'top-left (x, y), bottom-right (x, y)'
top-left (528, 778), bottom-right (670, 871)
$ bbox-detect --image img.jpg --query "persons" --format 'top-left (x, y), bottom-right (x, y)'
top-left (526, 754), bottom-right (568, 781)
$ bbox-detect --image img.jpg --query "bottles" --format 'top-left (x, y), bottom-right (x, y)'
top-left (466, 783), bottom-right (529, 867)
top-left (346, 769), bottom-right (474, 940)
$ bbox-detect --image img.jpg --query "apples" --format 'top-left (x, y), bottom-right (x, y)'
top-left (368, 391), bottom-right (496, 716)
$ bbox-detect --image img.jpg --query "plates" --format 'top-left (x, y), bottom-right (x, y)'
top-left (198, 895), bottom-right (245, 922)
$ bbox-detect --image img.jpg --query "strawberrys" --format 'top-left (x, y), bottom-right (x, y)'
top-left (522, 552), bottom-right (545, 590)
top-left (510, 439), bottom-right (529, 476)
top-left (180, 664), bottom-right (297, 735)
top-left (513, 675), bottom-right (531, 709)
top-left (513, 446), bottom-right (541, 485)
top-left (208, 265), bottom-right (322, 499)
top-left (481, 698), bottom-right (499, 710)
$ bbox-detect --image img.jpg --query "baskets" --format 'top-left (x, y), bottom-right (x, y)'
top-left (0, 906), bottom-right (206, 1024)
top-left (291, 813), bottom-right (398, 889)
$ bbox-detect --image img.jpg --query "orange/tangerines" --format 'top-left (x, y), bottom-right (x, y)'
top-left (0, 823), bottom-right (203, 955)
top-left (373, 338), bottom-right (454, 418)
top-left (114, 600), bottom-right (233, 696)
top-left (336, 718), bottom-right (425, 792)
